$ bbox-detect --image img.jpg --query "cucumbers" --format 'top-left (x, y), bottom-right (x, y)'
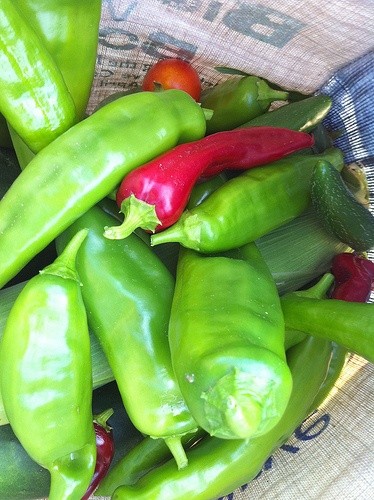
top-left (312, 161), bottom-right (374, 253)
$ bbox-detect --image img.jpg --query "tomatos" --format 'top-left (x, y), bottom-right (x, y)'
top-left (141, 59), bottom-right (201, 100)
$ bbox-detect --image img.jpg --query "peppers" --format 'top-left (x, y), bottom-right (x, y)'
top-left (0, 0), bottom-right (374, 500)
